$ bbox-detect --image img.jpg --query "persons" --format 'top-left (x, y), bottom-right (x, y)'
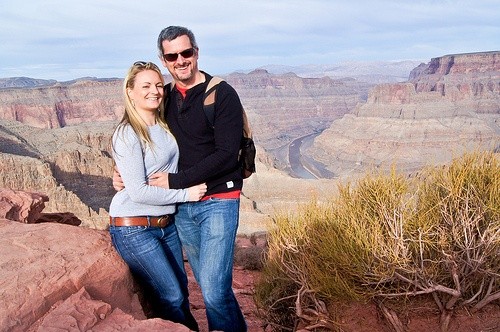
top-left (109, 60), bottom-right (208, 332)
top-left (112, 26), bottom-right (248, 332)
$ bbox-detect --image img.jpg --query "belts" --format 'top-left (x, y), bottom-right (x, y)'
top-left (110, 214), bottom-right (172, 228)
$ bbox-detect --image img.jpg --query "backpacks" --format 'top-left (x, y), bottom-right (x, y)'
top-left (169, 78), bottom-right (256, 178)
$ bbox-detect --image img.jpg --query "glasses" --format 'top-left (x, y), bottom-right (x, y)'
top-left (133, 61), bottom-right (160, 71)
top-left (163, 46), bottom-right (195, 62)
